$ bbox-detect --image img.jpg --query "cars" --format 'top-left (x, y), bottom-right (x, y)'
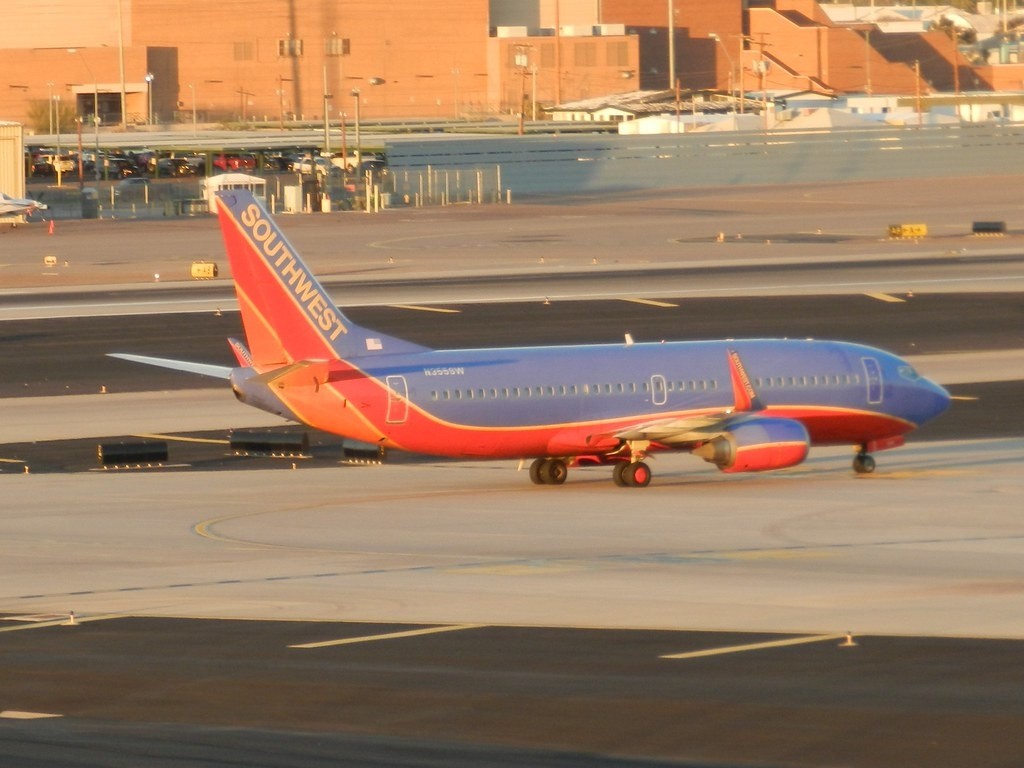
top-left (25, 146), bottom-right (389, 212)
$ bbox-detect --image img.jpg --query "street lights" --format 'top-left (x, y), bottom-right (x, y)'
top-left (351, 88), bottom-right (362, 182)
top-left (710, 33), bottom-right (738, 114)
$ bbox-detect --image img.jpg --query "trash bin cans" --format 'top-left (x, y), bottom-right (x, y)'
top-left (82, 188), bottom-right (100, 219)
top-left (304, 180), bottom-right (320, 210)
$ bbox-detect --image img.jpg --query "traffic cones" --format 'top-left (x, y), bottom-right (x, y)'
top-left (47, 220), bottom-right (56, 237)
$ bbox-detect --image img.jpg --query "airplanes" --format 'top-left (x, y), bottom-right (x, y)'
top-left (0, 192), bottom-right (49, 230)
top-left (106, 188), bottom-right (958, 489)
